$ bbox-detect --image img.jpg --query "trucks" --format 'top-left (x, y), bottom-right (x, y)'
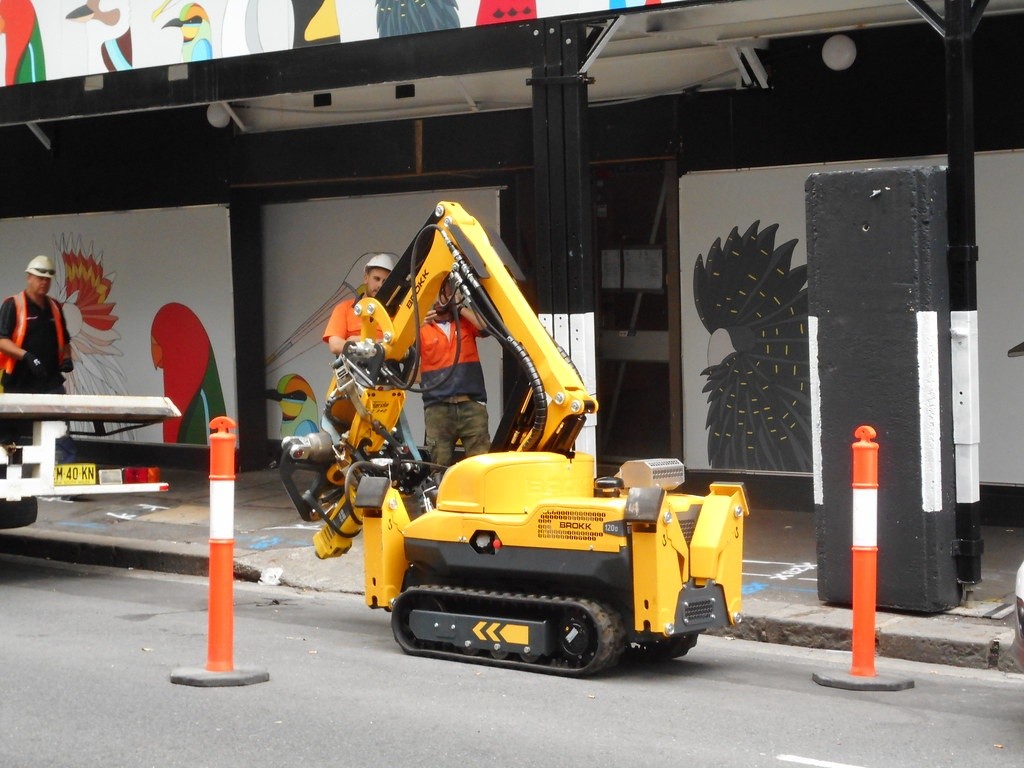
top-left (0, 392), bottom-right (183, 529)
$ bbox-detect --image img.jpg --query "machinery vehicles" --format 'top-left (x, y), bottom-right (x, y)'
top-left (278, 200), bottom-right (749, 680)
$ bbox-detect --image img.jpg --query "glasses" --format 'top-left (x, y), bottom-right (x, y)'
top-left (30, 267), bottom-right (55, 275)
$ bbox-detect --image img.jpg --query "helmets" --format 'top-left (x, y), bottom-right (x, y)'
top-left (25, 255), bottom-right (56, 278)
top-left (364, 253), bottom-right (399, 272)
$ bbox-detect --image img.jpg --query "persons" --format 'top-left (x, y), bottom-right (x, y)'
top-left (0, 255), bottom-right (82, 501)
top-left (420, 283), bottom-right (491, 474)
top-left (322, 254), bottom-right (398, 434)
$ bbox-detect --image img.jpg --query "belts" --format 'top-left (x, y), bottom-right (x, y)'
top-left (442, 395), bottom-right (470, 403)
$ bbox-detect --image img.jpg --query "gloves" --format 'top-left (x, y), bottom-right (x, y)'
top-left (59, 359), bottom-right (74, 372)
top-left (23, 351), bottom-right (47, 375)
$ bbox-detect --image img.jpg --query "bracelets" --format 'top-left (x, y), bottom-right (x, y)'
top-left (457, 304), bottom-right (468, 315)
